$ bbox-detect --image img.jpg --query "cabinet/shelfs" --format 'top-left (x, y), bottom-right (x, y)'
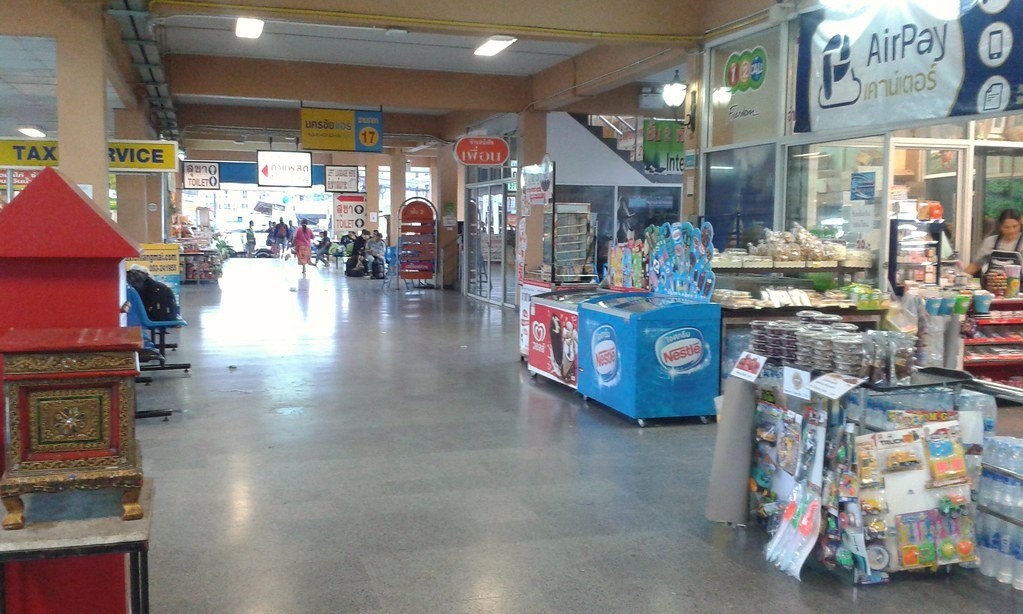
top-left (964, 298), bottom-right (1023, 394)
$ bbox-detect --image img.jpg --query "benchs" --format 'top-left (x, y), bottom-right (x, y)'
top-left (331, 241), bottom-right (398, 285)
top-left (127, 285), bottom-right (189, 423)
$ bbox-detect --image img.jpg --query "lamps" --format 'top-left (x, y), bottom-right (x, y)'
top-left (18, 125), bottom-right (48, 139)
top-left (474, 35), bottom-right (517, 57)
top-left (235, 18), bottom-right (264, 39)
top-left (662, 69), bottom-right (696, 131)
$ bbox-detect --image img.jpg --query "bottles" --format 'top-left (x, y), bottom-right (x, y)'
top-left (844, 386), bottom-right (1023, 593)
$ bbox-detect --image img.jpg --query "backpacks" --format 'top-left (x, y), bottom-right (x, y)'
top-left (277, 224), bottom-right (287, 238)
top-left (372, 258), bottom-right (386, 279)
top-left (141, 277), bottom-right (178, 321)
top-left (345, 256), bottom-right (365, 276)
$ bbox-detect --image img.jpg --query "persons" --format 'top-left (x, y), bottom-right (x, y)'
top-left (314, 230), bottom-right (386, 276)
top-left (245, 220), bottom-right (256, 255)
top-left (962, 209), bottom-right (1023, 294)
top-left (266, 217), bottom-right (298, 255)
top-left (292, 218), bottom-right (316, 273)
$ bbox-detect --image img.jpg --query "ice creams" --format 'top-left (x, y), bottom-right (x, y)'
top-left (549, 313), bottom-right (579, 378)
top-left (675, 237), bottom-right (713, 296)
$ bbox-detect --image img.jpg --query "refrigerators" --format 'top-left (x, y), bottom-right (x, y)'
top-left (519, 278), bottom-right (722, 426)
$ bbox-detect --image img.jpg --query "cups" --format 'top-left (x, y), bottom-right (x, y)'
top-left (926, 292), bottom-right (993, 314)
